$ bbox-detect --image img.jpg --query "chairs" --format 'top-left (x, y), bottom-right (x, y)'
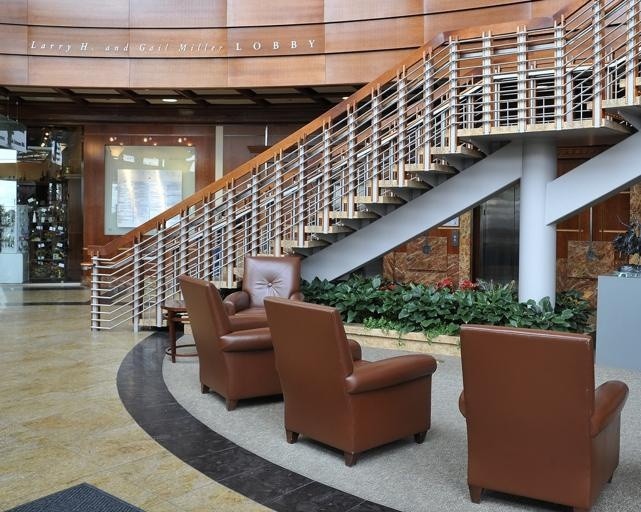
top-left (458, 325), bottom-right (629, 508)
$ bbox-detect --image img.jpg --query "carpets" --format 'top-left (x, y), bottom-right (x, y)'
top-left (0, 475), bottom-right (149, 510)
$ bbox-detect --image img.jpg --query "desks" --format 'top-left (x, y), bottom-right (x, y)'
top-left (161, 299), bottom-right (196, 361)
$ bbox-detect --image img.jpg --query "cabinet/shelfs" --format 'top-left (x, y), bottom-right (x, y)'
top-left (27, 177), bottom-right (67, 281)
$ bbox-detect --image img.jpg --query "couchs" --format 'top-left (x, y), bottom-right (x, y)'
top-left (179, 275), bottom-right (285, 412)
top-left (222, 258), bottom-right (304, 316)
top-left (262, 296), bottom-right (438, 467)
